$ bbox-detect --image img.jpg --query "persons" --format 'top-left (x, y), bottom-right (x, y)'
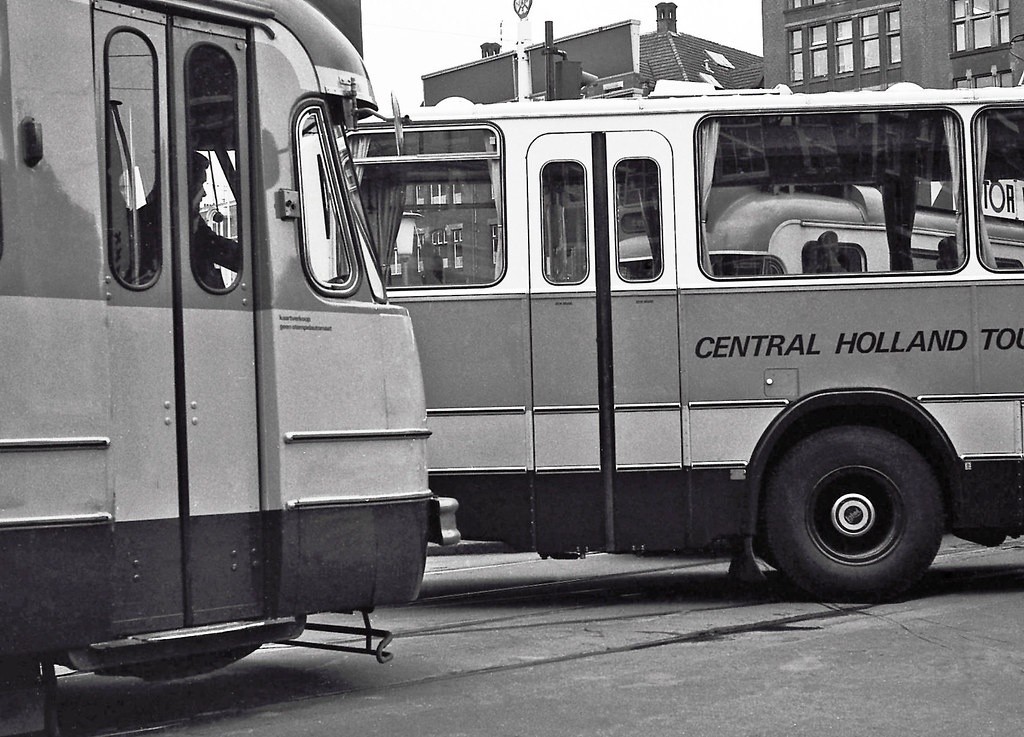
top-left (925, 236), bottom-right (958, 271)
top-left (133, 149), bottom-right (242, 289)
top-left (808, 229), bottom-right (846, 274)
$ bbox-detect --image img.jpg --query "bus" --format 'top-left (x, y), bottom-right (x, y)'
top-left (333, 81), bottom-right (1024, 605)
top-left (2, 1), bottom-right (461, 734)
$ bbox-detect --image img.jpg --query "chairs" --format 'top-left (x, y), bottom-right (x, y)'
top-left (617, 230), bottom-right (1023, 281)
